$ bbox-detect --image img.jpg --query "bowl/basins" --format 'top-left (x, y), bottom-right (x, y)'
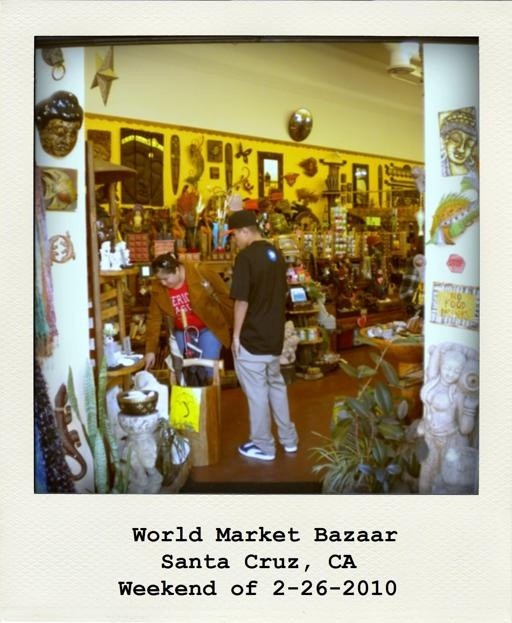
top-left (117, 390), bottom-right (157, 414)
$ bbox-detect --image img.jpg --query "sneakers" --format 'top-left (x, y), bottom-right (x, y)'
top-left (237, 441), bottom-right (276, 461)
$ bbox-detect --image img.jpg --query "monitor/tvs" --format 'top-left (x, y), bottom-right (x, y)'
top-left (289, 285), bottom-right (309, 304)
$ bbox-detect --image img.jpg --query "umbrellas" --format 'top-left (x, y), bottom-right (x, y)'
top-left (160, 305), bottom-right (213, 385)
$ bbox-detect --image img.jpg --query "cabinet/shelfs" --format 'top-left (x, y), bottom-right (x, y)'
top-left (86, 139), bottom-right (132, 393)
top-left (288, 305), bottom-right (323, 376)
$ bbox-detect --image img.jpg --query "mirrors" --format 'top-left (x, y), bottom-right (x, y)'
top-left (258, 151), bottom-right (282, 198)
top-left (353, 164), bottom-right (369, 208)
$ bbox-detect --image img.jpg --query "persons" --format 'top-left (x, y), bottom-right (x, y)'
top-left (218, 206), bottom-right (301, 460)
top-left (398, 235), bottom-right (426, 320)
top-left (437, 105), bottom-right (480, 175)
top-left (141, 250), bottom-right (235, 385)
top-left (35, 89), bottom-right (83, 159)
top-left (414, 338), bottom-right (478, 494)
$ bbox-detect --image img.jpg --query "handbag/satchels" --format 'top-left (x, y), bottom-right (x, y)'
top-left (192, 260), bottom-right (236, 329)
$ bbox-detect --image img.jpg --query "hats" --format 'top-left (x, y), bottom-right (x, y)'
top-left (218, 210), bottom-right (257, 238)
